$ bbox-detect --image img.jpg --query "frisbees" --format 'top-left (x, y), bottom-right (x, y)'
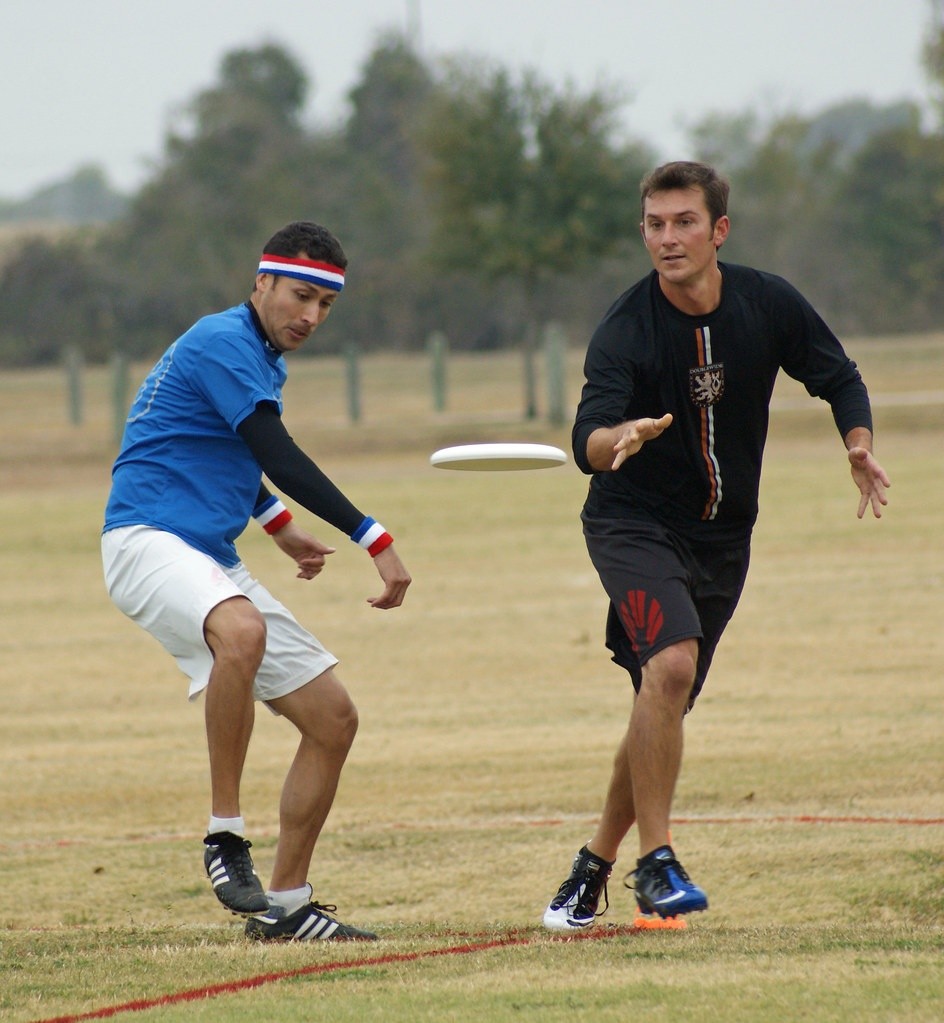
top-left (429, 440), bottom-right (569, 473)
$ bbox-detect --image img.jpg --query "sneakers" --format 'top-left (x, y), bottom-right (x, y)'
top-left (203, 831), bottom-right (271, 918)
top-left (244, 902), bottom-right (382, 945)
top-left (544, 839), bottom-right (617, 933)
top-left (623, 846), bottom-right (710, 921)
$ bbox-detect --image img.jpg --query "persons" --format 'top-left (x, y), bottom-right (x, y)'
top-left (543, 161), bottom-right (889, 930)
top-left (99, 224), bottom-right (413, 943)
